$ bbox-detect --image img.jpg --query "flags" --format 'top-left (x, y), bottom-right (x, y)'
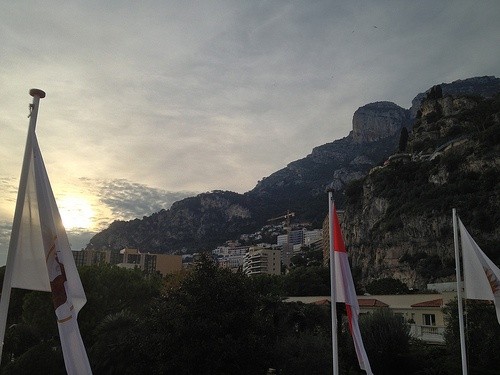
top-left (457, 217), bottom-right (500, 375)
top-left (0, 120), bottom-right (95, 375)
top-left (332, 198), bottom-right (375, 375)
